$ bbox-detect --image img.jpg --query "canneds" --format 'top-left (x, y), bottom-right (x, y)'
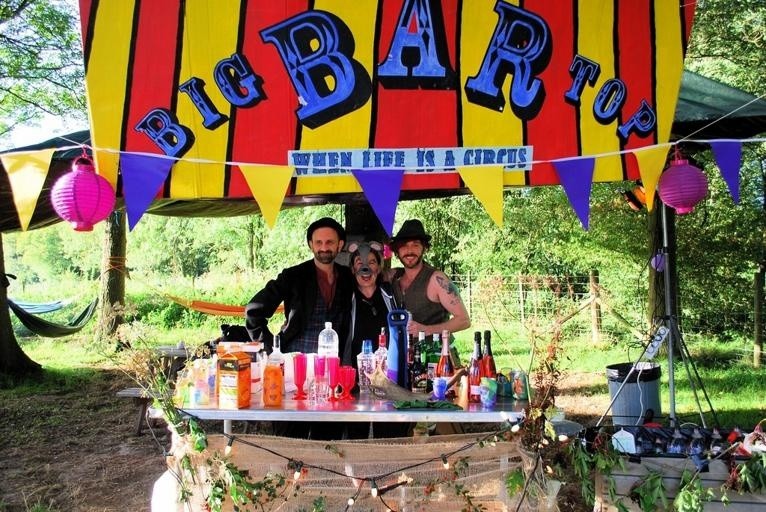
top-left (433, 379), bottom-right (446, 400)
top-left (511, 372), bottom-right (529, 400)
top-left (479, 380), bottom-right (499, 408)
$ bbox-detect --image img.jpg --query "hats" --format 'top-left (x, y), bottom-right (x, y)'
top-left (305, 216), bottom-right (347, 252)
top-left (388, 218), bottom-right (432, 245)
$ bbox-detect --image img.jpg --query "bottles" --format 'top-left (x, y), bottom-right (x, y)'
top-left (409, 329), bottom-right (497, 407)
top-left (263, 335), bottom-right (288, 406)
top-left (357, 328), bottom-right (388, 397)
top-left (318, 322), bottom-right (339, 386)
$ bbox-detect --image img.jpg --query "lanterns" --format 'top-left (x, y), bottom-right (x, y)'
top-left (659, 158), bottom-right (708, 214)
top-left (51, 164), bottom-right (116, 232)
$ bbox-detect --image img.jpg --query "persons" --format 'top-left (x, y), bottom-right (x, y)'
top-left (246, 217), bottom-right (405, 441)
top-left (376, 220), bottom-right (471, 436)
top-left (334, 241), bottom-right (408, 439)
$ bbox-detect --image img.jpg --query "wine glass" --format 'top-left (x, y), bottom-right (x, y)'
top-left (293, 354), bottom-right (356, 402)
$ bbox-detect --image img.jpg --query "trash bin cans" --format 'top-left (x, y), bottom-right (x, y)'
top-left (607, 362), bottom-right (661, 426)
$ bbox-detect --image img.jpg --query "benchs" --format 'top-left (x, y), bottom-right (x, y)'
top-left (116, 387), bottom-right (175, 436)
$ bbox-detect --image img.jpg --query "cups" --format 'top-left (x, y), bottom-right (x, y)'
top-left (176, 358), bottom-right (217, 407)
top-left (496, 367), bottom-right (529, 401)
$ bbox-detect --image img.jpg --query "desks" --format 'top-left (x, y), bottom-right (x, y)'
top-left (145, 347), bottom-right (190, 388)
top-left (147, 397), bottom-right (566, 512)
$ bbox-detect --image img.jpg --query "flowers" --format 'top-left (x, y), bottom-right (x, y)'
top-left (75, 299), bottom-right (262, 512)
